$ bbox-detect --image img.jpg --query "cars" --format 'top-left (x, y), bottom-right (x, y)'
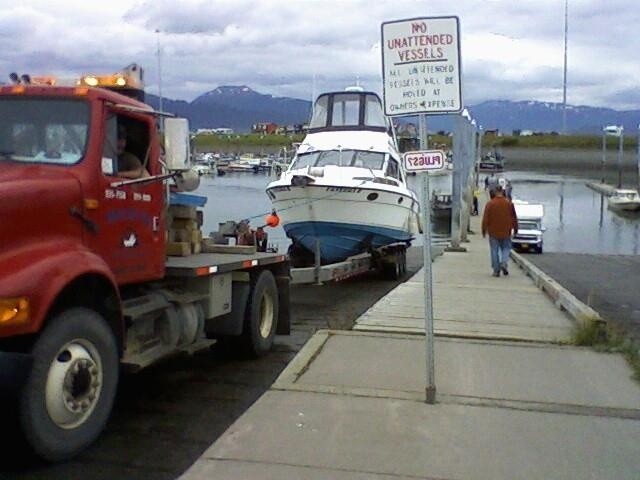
top-left (520, 130), bottom-right (560, 138)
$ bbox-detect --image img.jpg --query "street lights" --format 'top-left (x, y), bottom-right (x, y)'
top-left (154, 28), bottom-right (164, 133)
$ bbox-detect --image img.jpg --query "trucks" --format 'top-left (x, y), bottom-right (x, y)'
top-left (213, 127), bottom-right (236, 140)
top-left (605, 125), bottom-right (620, 138)
top-left (1, 61), bottom-right (293, 464)
top-left (512, 198), bottom-right (547, 253)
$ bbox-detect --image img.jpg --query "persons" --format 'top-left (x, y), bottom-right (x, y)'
top-left (484, 172), bottom-right (513, 202)
top-left (117, 123), bottom-right (151, 180)
top-left (481, 185), bottom-right (518, 278)
top-left (472, 184), bottom-right (480, 216)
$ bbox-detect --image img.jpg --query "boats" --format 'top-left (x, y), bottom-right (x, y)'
top-left (188, 148), bottom-right (290, 178)
top-left (429, 188), bottom-right (452, 219)
top-left (262, 73), bottom-right (424, 279)
top-left (606, 187), bottom-right (640, 212)
top-left (444, 145), bottom-right (506, 173)
